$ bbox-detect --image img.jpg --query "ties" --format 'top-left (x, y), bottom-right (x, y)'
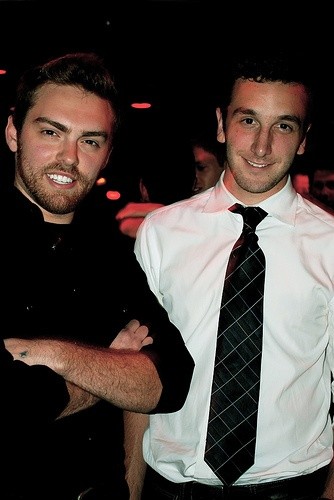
top-left (203, 204), bottom-right (266, 487)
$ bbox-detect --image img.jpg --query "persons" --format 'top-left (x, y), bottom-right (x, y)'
top-left (114, 156), bottom-right (166, 239)
top-left (189, 122), bottom-right (228, 194)
top-left (312, 159), bottom-right (334, 211)
top-left (0, 49), bottom-right (196, 500)
top-left (123, 53), bottom-right (334, 500)
